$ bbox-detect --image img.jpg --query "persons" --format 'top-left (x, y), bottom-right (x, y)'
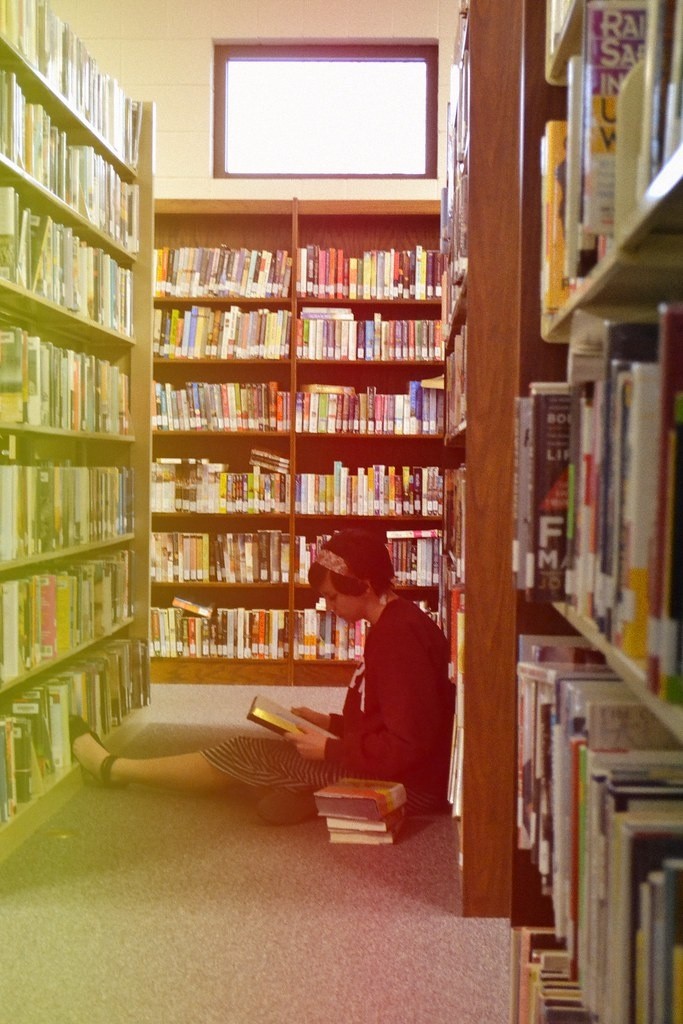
top-left (68, 525), bottom-right (456, 826)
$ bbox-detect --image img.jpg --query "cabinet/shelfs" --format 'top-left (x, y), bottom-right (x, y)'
top-left (150, 199), bottom-right (291, 686)
top-left (438, 0), bottom-right (546, 918)
top-left (508, 0), bottom-right (683, 1024)
top-left (0, 0), bottom-right (155, 867)
top-left (295, 200), bottom-right (443, 689)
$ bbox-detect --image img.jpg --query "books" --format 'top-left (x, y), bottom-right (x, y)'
top-left (311, 775), bottom-right (408, 847)
top-left (437, 0), bottom-right (683, 1023)
top-left (145, 238), bottom-right (450, 662)
top-left (0, 0), bottom-right (169, 847)
top-left (244, 692), bottom-right (341, 751)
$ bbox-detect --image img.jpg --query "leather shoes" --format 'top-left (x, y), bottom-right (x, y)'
top-left (257, 791), bottom-right (321, 824)
top-left (66, 713), bottom-right (127, 789)
